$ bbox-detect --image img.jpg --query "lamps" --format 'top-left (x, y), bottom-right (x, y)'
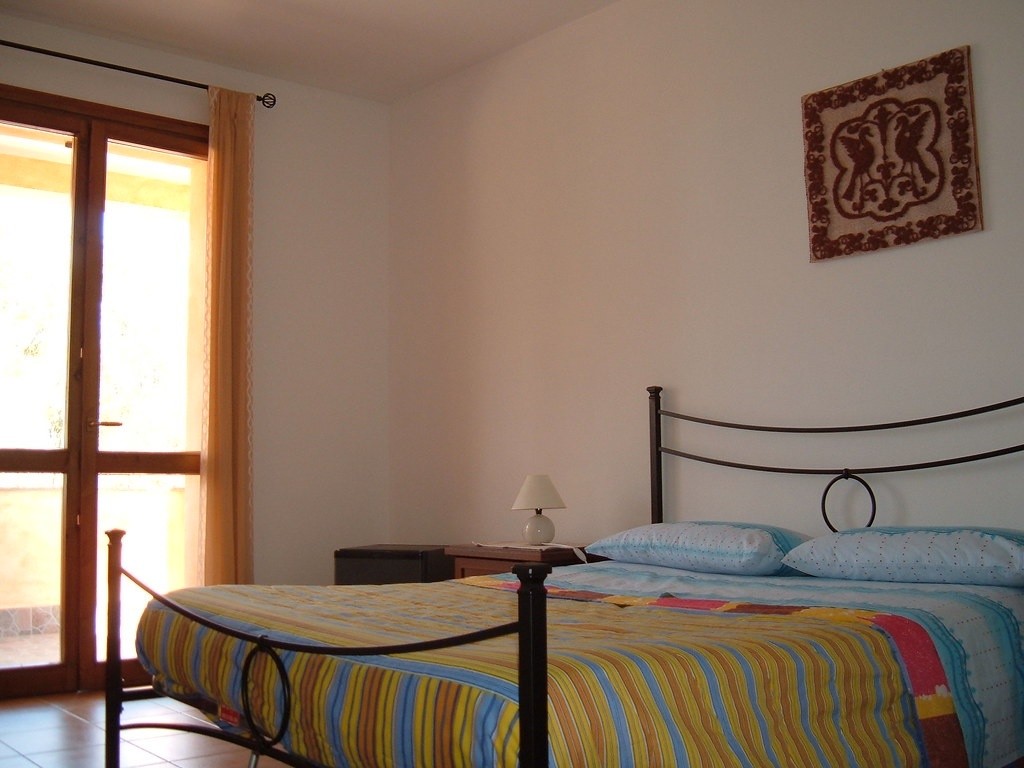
top-left (511, 474), bottom-right (567, 546)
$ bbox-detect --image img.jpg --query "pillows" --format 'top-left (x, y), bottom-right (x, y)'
top-left (586, 521), bottom-right (812, 575)
top-left (781, 526), bottom-right (1024, 588)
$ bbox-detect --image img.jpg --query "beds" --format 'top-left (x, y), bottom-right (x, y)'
top-left (104, 386), bottom-right (1024, 768)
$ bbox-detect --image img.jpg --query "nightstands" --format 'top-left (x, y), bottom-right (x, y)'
top-left (444, 543), bottom-right (597, 580)
top-left (333, 544), bottom-right (454, 586)
